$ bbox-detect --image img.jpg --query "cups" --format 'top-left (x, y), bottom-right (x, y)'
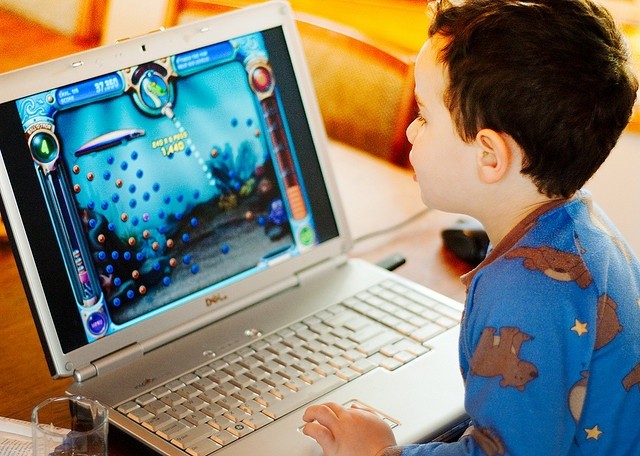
top-left (33, 394), bottom-right (108, 455)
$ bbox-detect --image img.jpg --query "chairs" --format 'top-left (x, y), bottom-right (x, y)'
top-left (159, 0), bottom-right (416, 166)
top-left (1, 0), bottom-right (111, 44)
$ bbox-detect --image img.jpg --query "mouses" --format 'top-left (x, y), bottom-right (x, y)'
top-left (440, 216), bottom-right (489, 265)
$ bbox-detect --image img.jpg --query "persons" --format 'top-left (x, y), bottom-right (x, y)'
top-left (303, 0), bottom-right (640, 455)
